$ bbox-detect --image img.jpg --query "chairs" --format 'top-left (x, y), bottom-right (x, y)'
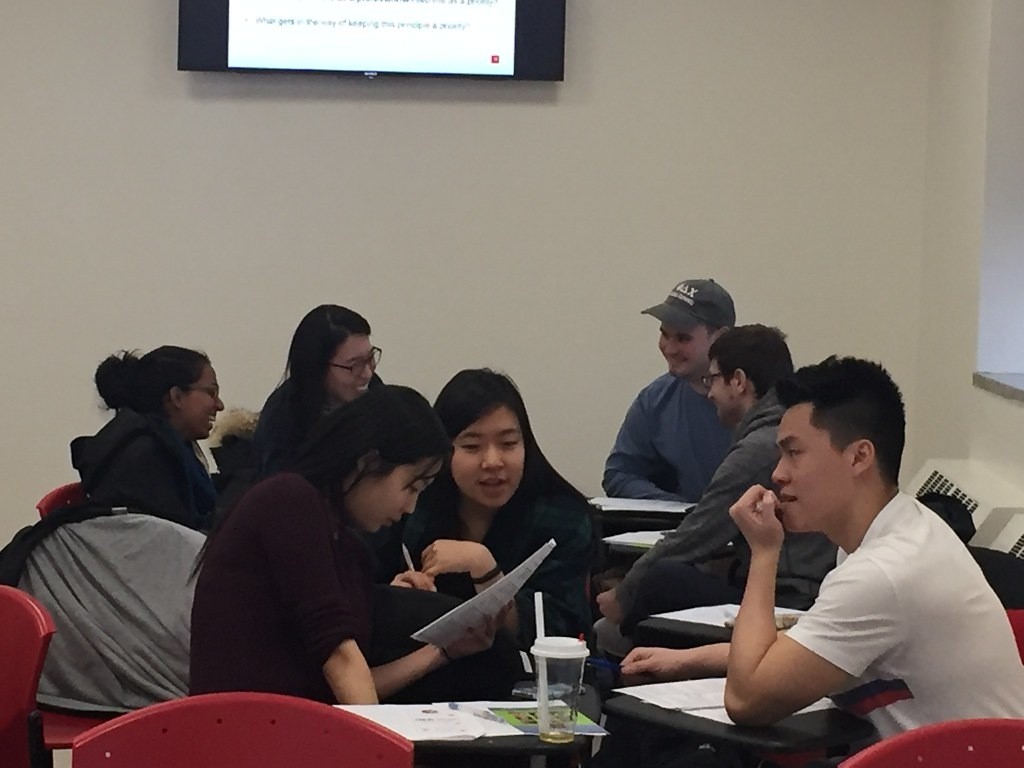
top-left (72, 691), bottom-right (414, 768)
top-left (0, 585), bottom-right (57, 768)
top-left (837, 718), bottom-right (1024, 768)
top-left (35, 482), bottom-right (86, 519)
top-left (16, 515), bottom-right (210, 768)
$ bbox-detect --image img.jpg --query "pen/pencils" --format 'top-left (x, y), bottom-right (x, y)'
top-left (402, 543), bottom-right (415, 572)
top-left (449, 702), bottom-right (504, 723)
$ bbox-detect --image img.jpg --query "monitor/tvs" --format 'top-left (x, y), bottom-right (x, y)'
top-left (177, 0), bottom-right (567, 83)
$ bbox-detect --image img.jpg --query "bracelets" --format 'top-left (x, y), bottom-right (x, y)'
top-left (470, 563), bottom-right (502, 584)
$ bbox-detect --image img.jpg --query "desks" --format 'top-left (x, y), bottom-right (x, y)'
top-left (606, 528), bottom-right (734, 563)
top-left (634, 604), bottom-right (808, 648)
top-left (331, 702), bottom-right (591, 768)
top-left (603, 674), bottom-right (878, 768)
top-left (586, 495), bottom-right (695, 534)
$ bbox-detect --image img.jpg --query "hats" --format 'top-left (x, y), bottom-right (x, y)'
top-left (642, 279), bottom-right (736, 332)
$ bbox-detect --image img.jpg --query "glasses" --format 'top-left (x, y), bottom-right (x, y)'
top-left (327, 346), bottom-right (382, 377)
top-left (185, 386), bottom-right (220, 400)
top-left (702, 373), bottom-right (723, 391)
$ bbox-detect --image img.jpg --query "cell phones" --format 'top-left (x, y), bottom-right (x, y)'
top-left (512, 683), bottom-right (585, 699)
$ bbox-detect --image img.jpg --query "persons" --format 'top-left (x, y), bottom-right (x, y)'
top-left (369, 367), bottom-right (600, 768)
top-left (252, 303), bottom-right (384, 463)
top-left (188, 384), bottom-right (515, 705)
top-left (619, 357), bottom-right (1024, 768)
top-left (595, 324), bottom-right (838, 768)
top-left (601, 279), bottom-right (736, 502)
top-left (70, 346), bottom-right (225, 533)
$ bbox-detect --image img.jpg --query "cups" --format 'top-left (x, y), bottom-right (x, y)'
top-left (530, 637), bottom-right (590, 743)
